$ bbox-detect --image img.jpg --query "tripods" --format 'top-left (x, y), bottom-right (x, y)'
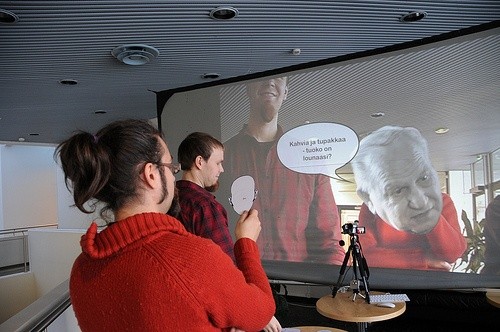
top-left (332, 236), bottom-right (370, 304)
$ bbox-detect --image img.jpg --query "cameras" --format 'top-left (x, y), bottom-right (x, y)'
top-left (341, 220), bottom-right (365, 235)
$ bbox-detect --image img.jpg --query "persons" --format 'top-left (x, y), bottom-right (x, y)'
top-left (167, 132), bottom-right (282, 332)
top-left (347, 126), bottom-right (467, 272)
top-left (53, 118), bottom-right (275, 332)
top-left (215, 73), bottom-right (350, 265)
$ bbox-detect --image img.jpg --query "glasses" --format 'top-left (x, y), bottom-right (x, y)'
top-left (139, 161), bottom-right (181, 174)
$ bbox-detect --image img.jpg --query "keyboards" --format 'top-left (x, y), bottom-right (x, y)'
top-left (368, 293), bottom-right (411, 303)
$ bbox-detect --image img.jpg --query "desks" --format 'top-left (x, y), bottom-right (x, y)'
top-left (316, 291), bottom-right (406, 332)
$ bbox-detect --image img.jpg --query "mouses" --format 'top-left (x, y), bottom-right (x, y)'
top-left (376, 302), bottom-right (396, 308)
